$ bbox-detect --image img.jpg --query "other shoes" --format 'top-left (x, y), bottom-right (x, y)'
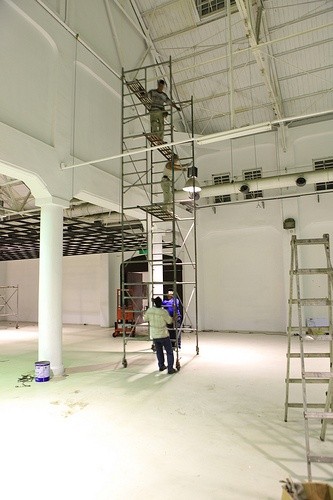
top-left (159, 366), bottom-right (166, 371)
top-left (168, 369), bottom-right (177, 374)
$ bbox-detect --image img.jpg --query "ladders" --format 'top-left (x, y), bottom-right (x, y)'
top-left (112, 55), bottom-right (208, 372)
top-left (284, 233), bottom-right (333, 482)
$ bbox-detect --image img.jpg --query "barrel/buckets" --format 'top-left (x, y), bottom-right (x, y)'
top-left (34, 360), bottom-right (50, 383)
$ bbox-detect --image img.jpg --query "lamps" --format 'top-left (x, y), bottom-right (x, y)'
top-left (183, 167), bottom-right (201, 193)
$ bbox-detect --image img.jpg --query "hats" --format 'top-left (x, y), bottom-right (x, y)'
top-left (155, 297), bottom-right (163, 307)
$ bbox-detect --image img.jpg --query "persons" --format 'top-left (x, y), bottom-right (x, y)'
top-left (148, 80), bottom-right (181, 139)
top-left (143, 297), bottom-right (176, 374)
top-left (161, 154), bottom-right (187, 211)
top-left (162, 289), bottom-right (182, 350)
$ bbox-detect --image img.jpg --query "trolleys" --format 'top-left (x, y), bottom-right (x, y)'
top-left (113, 289), bottom-right (136, 337)
